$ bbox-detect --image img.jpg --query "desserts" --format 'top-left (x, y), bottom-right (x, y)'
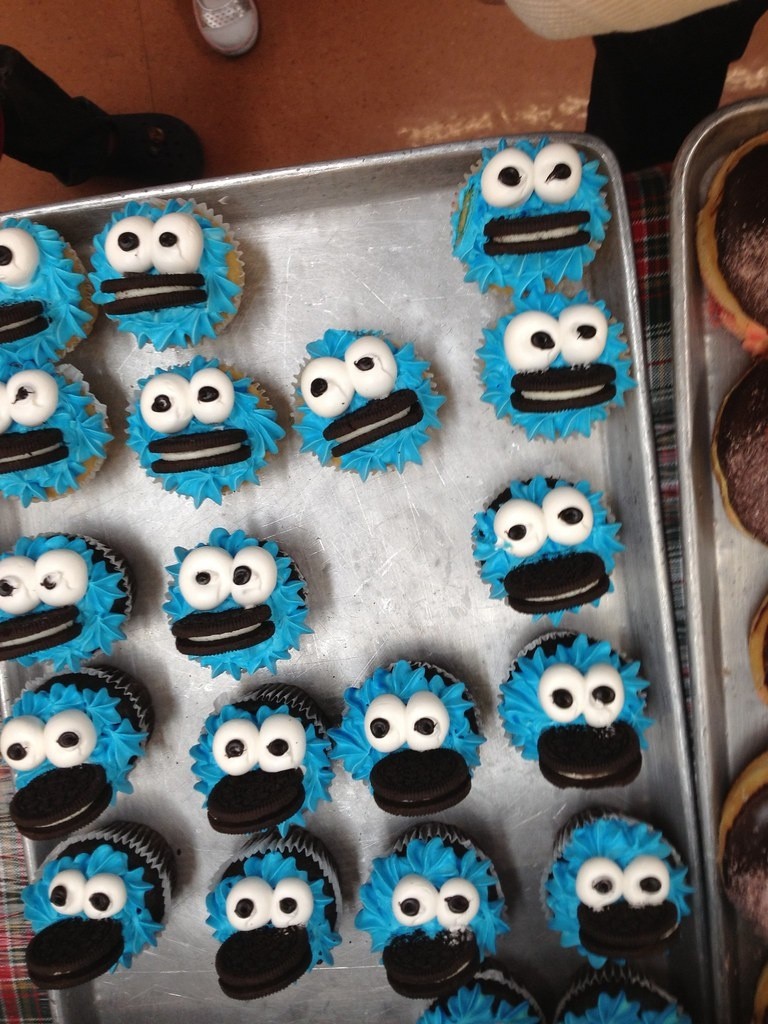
top-left (0, 130), bottom-right (768, 1024)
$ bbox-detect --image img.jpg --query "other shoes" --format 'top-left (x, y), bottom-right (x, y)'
top-left (51, 95), bottom-right (205, 187)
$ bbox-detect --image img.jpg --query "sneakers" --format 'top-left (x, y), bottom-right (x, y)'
top-left (192, 0), bottom-right (258, 56)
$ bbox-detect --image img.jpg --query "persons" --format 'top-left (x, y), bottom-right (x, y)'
top-left (0, 44), bottom-right (204, 182)
